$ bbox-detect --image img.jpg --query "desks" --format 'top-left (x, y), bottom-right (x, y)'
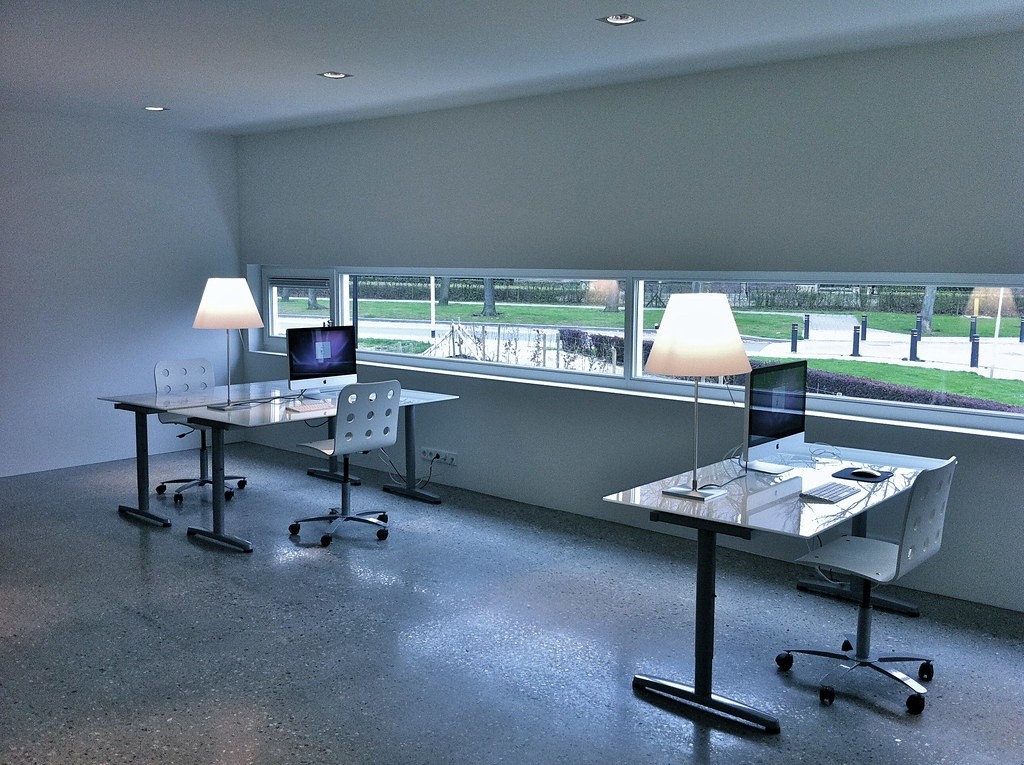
top-left (166, 374), bottom-right (460, 552)
top-left (97, 380), bottom-right (365, 527)
top-left (601, 442), bottom-right (959, 739)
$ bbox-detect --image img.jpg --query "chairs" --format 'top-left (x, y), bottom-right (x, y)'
top-left (154, 357), bottom-right (247, 503)
top-left (288, 380), bottom-right (401, 546)
top-left (775, 455), bottom-right (957, 717)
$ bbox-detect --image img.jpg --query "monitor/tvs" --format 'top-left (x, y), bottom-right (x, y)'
top-left (739, 360), bottom-right (807, 474)
top-left (286, 325), bottom-right (357, 400)
top-left (743, 471), bottom-right (802, 535)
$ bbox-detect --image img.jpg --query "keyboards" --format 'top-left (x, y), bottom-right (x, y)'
top-left (285, 403), bottom-right (336, 413)
top-left (799, 481), bottom-right (861, 504)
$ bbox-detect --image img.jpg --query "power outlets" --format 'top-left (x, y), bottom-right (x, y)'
top-left (419, 447), bottom-right (457, 466)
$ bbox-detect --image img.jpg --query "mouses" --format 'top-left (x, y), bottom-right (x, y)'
top-left (851, 469), bottom-right (881, 478)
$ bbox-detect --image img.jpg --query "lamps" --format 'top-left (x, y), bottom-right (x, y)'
top-left (644, 292), bottom-right (752, 502)
top-left (192, 277), bottom-right (264, 411)
